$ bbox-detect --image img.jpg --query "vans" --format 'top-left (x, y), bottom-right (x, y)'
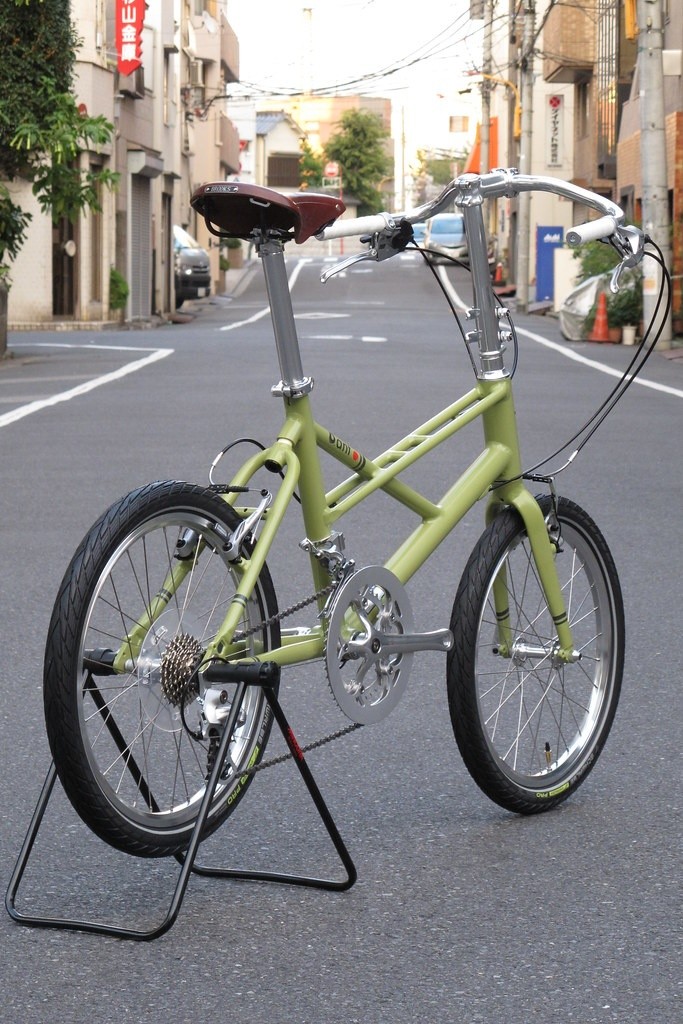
top-left (173, 225), bottom-right (211, 308)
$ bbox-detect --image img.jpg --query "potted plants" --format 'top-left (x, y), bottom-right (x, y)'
top-left (607, 290), bottom-right (645, 345)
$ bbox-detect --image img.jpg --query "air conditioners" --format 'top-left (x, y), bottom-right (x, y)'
top-left (191, 60), bottom-right (203, 85)
top-left (118, 65), bottom-right (145, 99)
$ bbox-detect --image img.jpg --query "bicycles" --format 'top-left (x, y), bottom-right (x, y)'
top-left (4, 169), bottom-right (672, 940)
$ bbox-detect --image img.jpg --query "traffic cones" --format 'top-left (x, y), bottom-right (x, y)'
top-left (583, 291), bottom-right (612, 340)
top-left (494, 262), bottom-right (503, 282)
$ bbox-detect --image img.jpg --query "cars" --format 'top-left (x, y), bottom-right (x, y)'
top-left (405, 213), bottom-right (470, 265)
